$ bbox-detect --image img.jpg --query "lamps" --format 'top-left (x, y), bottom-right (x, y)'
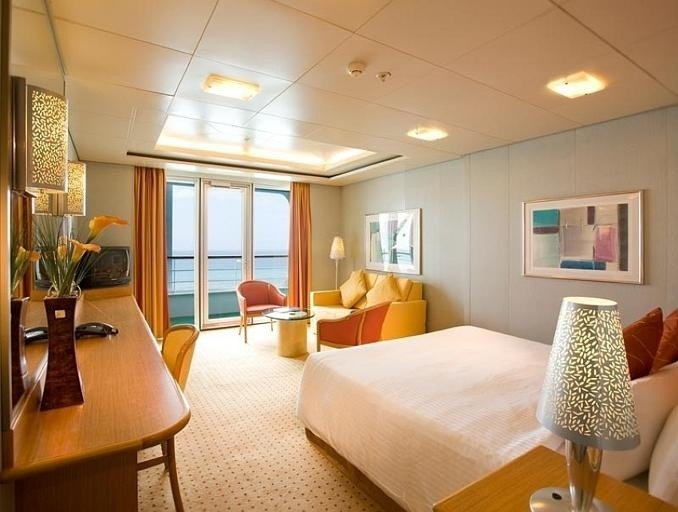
top-left (527, 294), bottom-right (642, 512)
top-left (56, 159), bottom-right (87, 217)
top-left (329, 237), bottom-right (345, 289)
top-left (407, 126), bottom-right (447, 142)
top-left (204, 75), bottom-right (260, 103)
top-left (12, 75), bottom-right (69, 199)
top-left (545, 70), bottom-right (607, 99)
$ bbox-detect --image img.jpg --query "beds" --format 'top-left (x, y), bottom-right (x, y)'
top-left (293, 304), bottom-right (678, 512)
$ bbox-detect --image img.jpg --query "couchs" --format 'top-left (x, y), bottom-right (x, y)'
top-left (308, 270), bottom-right (426, 347)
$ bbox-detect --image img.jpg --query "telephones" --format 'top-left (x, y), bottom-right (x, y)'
top-left (23, 327), bottom-right (49, 345)
top-left (75, 322), bottom-right (118, 341)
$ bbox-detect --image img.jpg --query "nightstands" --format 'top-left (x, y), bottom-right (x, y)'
top-left (432, 444), bottom-right (678, 512)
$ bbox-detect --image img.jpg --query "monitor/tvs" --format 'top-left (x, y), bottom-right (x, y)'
top-left (80, 246), bottom-right (132, 287)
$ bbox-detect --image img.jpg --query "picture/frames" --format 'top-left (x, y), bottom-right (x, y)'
top-left (364, 208), bottom-right (422, 276)
top-left (522, 189), bottom-right (645, 286)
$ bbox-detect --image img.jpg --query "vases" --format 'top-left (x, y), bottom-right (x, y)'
top-left (10, 296), bottom-right (33, 409)
top-left (40, 293), bottom-right (85, 411)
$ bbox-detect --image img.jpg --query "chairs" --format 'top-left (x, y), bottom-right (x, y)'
top-left (317, 300), bottom-right (393, 352)
top-left (236, 280), bottom-right (288, 343)
top-left (136, 324), bottom-right (199, 511)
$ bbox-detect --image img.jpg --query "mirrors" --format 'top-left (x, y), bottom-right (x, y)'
top-left (9, 186), bottom-right (71, 413)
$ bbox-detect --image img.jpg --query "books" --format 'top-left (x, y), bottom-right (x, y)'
top-left (272, 305), bottom-right (299, 312)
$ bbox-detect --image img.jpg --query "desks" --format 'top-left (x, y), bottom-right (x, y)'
top-left (10, 285), bottom-right (191, 511)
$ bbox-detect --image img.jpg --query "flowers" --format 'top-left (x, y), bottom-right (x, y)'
top-left (9, 216), bottom-right (41, 296)
top-left (32, 206), bottom-right (131, 297)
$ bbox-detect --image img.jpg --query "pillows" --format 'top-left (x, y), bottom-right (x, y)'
top-left (365, 273), bottom-right (402, 308)
top-left (340, 269), bottom-right (367, 308)
top-left (622, 306), bottom-right (663, 380)
top-left (649, 307), bottom-right (678, 375)
top-left (583, 361), bottom-right (678, 481)
